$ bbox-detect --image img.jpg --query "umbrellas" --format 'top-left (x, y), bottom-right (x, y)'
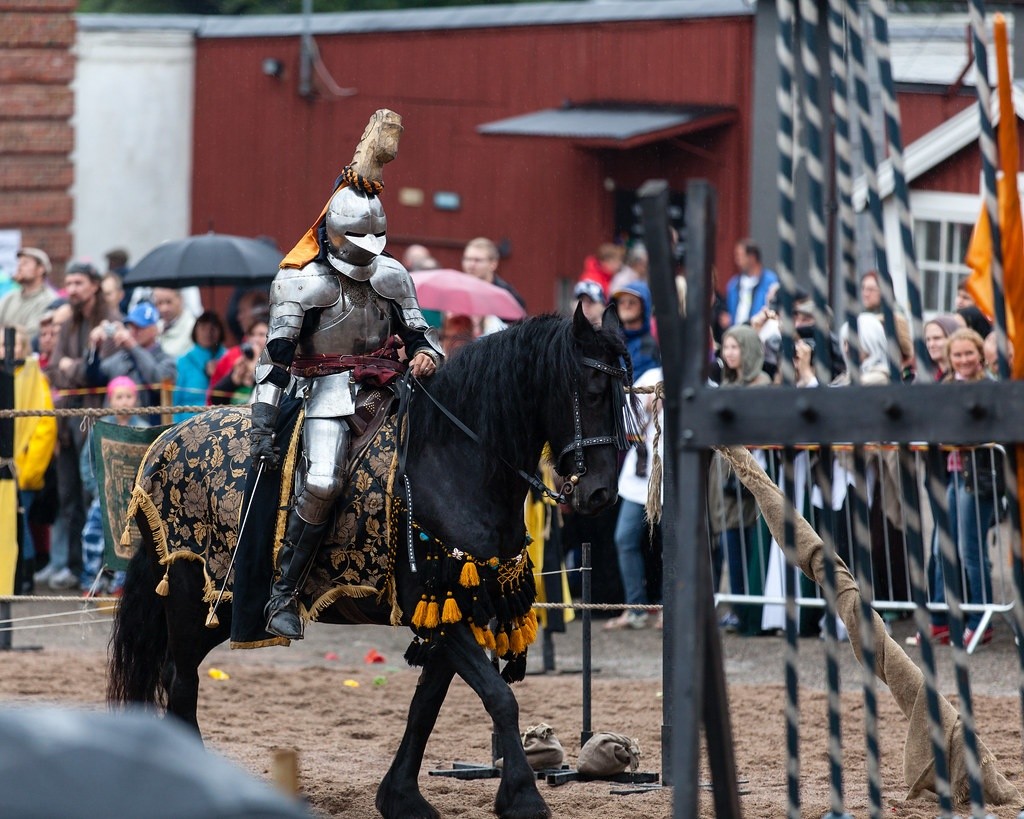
top-left (410, 268), bottom-right (526, 320)
top-left (117, 224), bottom-right (286, 308)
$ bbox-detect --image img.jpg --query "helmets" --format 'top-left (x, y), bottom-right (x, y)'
top-left (324, 184), bottom-right (388, 281)
top-left (611, 264), bottom-right (651, 319)
top-left (573, 255), bottom-right (609, 306)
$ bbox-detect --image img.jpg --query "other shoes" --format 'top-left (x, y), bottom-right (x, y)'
top-left (724, 620), bottom-right (738, 632)
top-left (105, 589), bottom-right (124, 598)
top-left (34, 563), bottom-right (57, 584)
top-left (84, 590), bottom-right (100, 598)
top-left (50, 568), bottom-right (79, 591)
top-left (603, 610), bottom-right (651, 629)
top-left (654, 610), bottom-right (664, 628)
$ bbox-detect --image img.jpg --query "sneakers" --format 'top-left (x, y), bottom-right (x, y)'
top-left (950, 626), bottom-right (995, 651)
top-left (906, 623), bottom-right (949, 645)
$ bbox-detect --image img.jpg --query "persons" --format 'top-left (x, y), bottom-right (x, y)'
top-left (0, 248), bottom-right (270, 602)
top-left (407, 238), bottom-right (524, 353)
top-left (249, 185), bottom-right (445, 639)
top-left (573, 244), bottom-right (1011, 648)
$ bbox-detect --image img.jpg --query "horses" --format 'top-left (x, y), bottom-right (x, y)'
top-left (106, 299), bottom-right (646, 819)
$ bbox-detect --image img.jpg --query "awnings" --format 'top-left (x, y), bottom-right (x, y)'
top-left (477, 100), bottom-right (739, 157)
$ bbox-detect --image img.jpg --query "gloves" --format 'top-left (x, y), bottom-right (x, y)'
top-left (249, 402), bottom-right (287, 471)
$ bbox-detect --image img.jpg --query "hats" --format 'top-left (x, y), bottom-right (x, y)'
top-left (121, 304), bottom-right (159, 329)
top-left (925, 317), bottom-right (960, 339)
top-left (64, 263), bottom-right (103, 294)
top-left (17, 246), bottom-right (52, 276)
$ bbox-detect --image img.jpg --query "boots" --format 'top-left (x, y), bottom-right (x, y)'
top-left (265, 506), bottom-right (331, 639)
